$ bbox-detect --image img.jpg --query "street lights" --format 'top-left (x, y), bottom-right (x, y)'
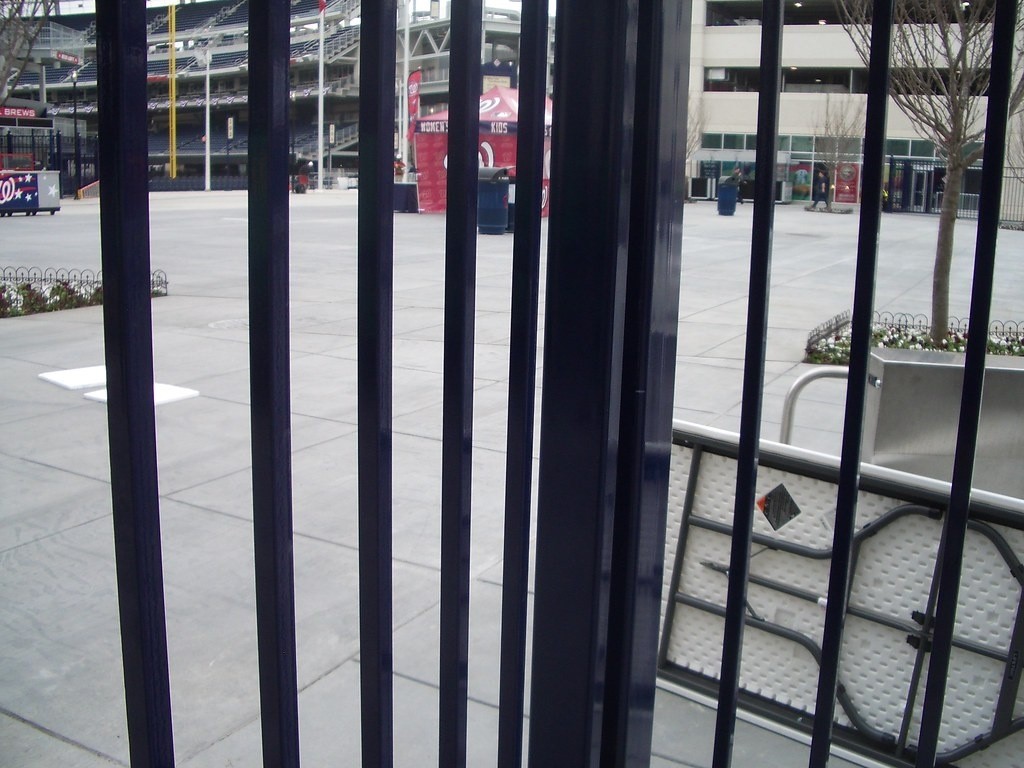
top-left (195, 49), bottom-right (212, 191)
top-left (71, 70), bottom-right (83, 188)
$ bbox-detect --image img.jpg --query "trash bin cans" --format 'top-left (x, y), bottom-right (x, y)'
top-left (717, 175), bottom-right (737, 216)
top-left (475, 167), bottom-right (510, 236)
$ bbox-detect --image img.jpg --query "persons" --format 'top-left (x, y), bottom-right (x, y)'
top-left (394, 154), bottom-right (406, 182)
top-left (810, 170), bottom-right (830, 210)
top-left (732, 166), bottom-right (747, 204)
top-left (883, 184), bottom-right (889, 209)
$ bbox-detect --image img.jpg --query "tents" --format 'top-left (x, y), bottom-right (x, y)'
top-left (413, 85), bottom-right (553, 217)
